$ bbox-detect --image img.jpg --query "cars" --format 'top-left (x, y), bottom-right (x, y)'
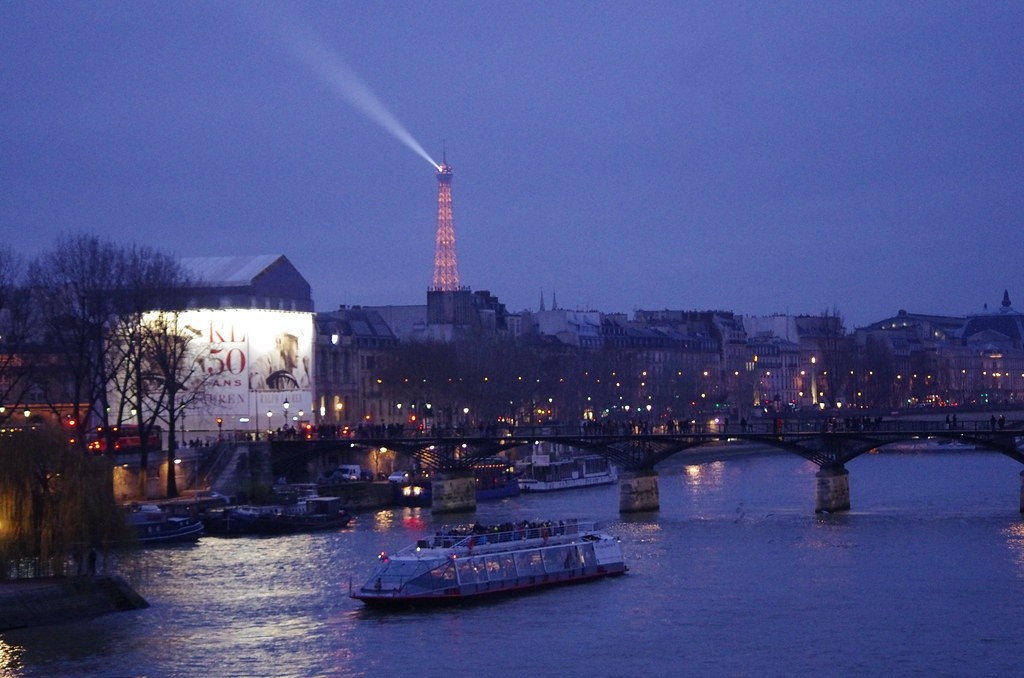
top-left (388, 471), bottom-right (410, 483)
top-left (197, 491), bottom-right (230, 507)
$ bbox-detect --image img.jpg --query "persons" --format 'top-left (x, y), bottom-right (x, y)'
top-left (357, 423), bottom-right (403, 438)
top-left (249, 334), bottom-right (311, 391)
top-left (844, 413), bottom-right (883, 432)
top-left (741, 416), bottom-right (753, 433)
top-left (276, 423), bottom-right (338, 440)
top-left (432, 520), bottom-right (565, 545)
top-left (724, 417), bottom-right (730, 433)
top-left (583, 416), bottom-right (689, 435)
top-left (176, 437), bottom-right (209, 447)
top-left (990, 414), bottom-right (1005, 432)
top-left (773, 417), bottom-right (784, 433)
top-left (479, 421), bottom-right (514, 437)
top-left (946, 414), bottom-right (957, 430)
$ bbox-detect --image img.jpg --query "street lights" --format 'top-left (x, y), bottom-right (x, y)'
top-left (267, 410), bottom-right (272, 431)
top-left (283, 398), bottom-right (289, 424)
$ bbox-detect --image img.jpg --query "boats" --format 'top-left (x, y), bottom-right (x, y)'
top-left (517, 455), bottom-right (619, 491)
top-left (348, 518), bottom-right (630, 609)
top-left (399, 475), bottom-right (520, 507)
top-left (122, 504), bottom-right (204, 546)
top-left (222, 496), bottom-right (353, 535)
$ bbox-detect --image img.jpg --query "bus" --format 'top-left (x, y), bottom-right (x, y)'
top-left (97, 424), bottom-right (161, 453)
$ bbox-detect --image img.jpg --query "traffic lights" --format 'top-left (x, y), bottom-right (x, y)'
top-left (218, 418), bottom-right (222, 427)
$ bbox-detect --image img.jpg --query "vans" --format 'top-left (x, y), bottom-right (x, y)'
top-left (338, 465), bottom-right (359, 479)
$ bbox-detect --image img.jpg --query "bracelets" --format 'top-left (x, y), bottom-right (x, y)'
top-left (250, 372), bottom-right (258, 377)
